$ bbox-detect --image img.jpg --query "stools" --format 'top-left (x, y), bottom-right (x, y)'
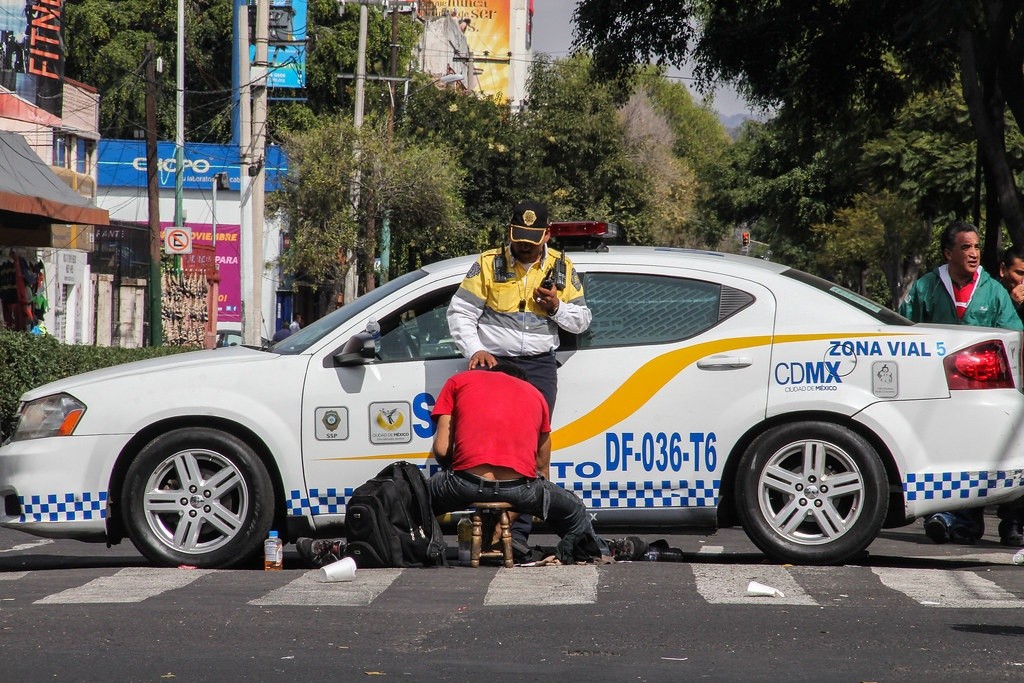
top-left (468, 501), bottom-right (515, 569)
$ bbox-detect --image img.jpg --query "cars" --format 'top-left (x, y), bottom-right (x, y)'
top-left (0, 221), bottom-right (1024, 562)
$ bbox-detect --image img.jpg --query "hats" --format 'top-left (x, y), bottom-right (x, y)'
top-left (511, 198), bottom-right (552, 246)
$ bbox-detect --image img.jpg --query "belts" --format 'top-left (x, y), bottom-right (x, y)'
top-left (453, 469), bottom-right (535, 488)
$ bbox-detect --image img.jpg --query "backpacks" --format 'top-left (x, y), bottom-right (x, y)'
top-left (345, 461), bottom-right (450, 569)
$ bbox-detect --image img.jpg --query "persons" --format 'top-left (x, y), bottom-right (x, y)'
top-left (289, 312), bottom-right (301, 335)
top-left (299, 315), bottom-right (308, 329)
top-left (273, 320), bottom-right (294, 345)
top-left (446, 200), bottom-right (593, 556)
top-left (427, 364), bottom-right (645, 562)
top-left (897, 221), bottom-right (1024, 544)
top-left (996, 245), bottom-right (1024, 546)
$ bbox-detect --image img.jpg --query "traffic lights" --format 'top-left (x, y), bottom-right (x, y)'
top-left (742, 231), bottom-right (750, 247)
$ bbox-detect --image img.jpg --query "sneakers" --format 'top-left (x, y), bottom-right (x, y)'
top-left (297, 536), bottom-right (344, 568)
top-left (605, 534), bottom-right (648, 561)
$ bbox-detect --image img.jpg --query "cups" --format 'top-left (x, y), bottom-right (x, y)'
top-left (319, 557), bottom-right (358, 582)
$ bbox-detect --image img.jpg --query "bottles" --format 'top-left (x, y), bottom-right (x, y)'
top-left (641, 546), bottom-right (683, 562)
top-left (457, 509), bottom-right (475, 565)
top-left (366, 317), bottom-right (381, 355)
top-left (264, 531), bottom-right (283, 572)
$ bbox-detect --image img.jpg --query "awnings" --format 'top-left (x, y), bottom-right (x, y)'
top-left (0, 130), bottom-right (110, 225)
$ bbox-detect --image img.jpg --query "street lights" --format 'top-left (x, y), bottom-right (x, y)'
top-left (379, 73), bottom-right (464, 285)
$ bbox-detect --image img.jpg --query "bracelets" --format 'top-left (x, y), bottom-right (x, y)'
top-left (549, 307), bottom-right (559, 316)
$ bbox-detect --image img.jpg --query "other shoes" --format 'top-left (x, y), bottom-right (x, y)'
top-left (924, 516), bottom-right (951, 544)
top-left (508, 549), bottom-right (554, 568)
top-left (641, 539), bottom-right (684, 562)
top-left (951, 529), bottom-right (974, 545)
top-left (999, 521), bottom-right (1023, 546)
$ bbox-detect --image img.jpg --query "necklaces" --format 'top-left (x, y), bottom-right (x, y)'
top-left (514, 266), bottom-right (539, 312)
